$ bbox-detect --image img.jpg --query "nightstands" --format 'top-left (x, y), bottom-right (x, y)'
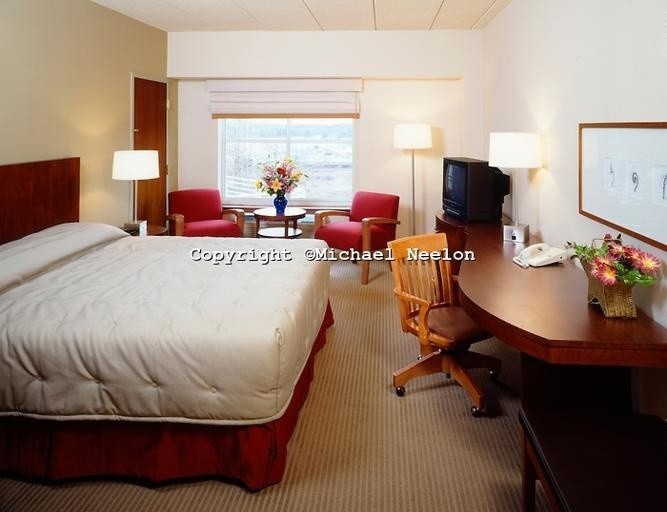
top-left (120, 224), bottom-right (167, 237)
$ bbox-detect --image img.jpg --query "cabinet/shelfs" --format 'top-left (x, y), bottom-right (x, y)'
top-left (514, 400), bottom-right (667, 512)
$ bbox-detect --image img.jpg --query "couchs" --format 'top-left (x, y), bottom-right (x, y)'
top-left (167, 188), bottom-right (246, 238)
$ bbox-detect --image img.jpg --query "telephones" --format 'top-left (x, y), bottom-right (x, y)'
top-left (512, 243), bottom-right (565, 269)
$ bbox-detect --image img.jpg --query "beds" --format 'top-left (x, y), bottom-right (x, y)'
top-left (0, 157), bottom-right (332, 491)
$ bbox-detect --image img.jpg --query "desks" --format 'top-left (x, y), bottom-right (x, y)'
top-left (435, 210), bottom-right (667, 394)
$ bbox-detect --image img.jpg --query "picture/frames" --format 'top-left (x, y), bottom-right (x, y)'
top-left (578, 122), bottom-right (667, 253)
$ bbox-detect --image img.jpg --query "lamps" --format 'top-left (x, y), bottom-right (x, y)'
top-left (110, 149), bottom-right (160, 234)
top-left (487, 130), bottom-right (545, 243)
top-left (392, 123), bottom-right (434, 237)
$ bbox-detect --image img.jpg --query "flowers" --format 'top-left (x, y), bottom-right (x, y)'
top-left (571, 232), bottom-right (662, 285)
top-left (250, 154), bottom-right (311, 196)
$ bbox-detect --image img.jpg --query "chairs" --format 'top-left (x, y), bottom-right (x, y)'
top-left (314, 191), bottom-right (400, 285)
top-left (385, 231), bottom-right (519, 419)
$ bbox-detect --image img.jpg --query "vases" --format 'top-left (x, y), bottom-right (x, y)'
top-left (579, 259), bottom-right (638, 319)
top-left (273, 196), bottom-right (288, 214)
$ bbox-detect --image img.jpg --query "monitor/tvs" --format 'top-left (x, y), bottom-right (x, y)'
top-left (442, 157), bottom-right (510, 223)
